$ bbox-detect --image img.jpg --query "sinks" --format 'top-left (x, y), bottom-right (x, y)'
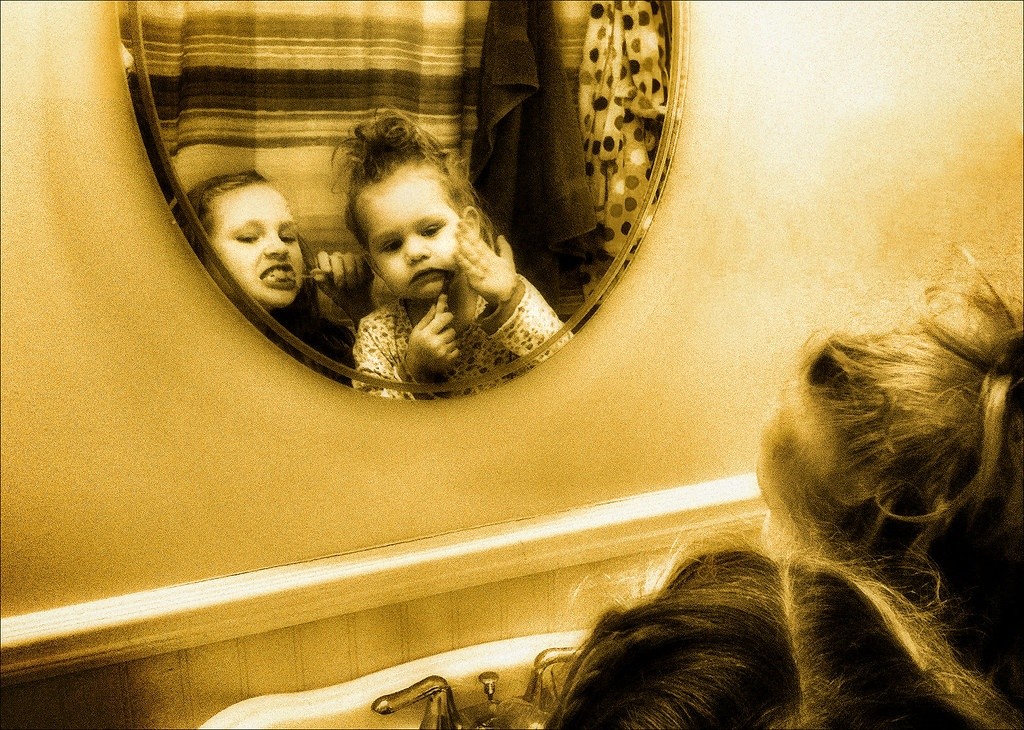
top-left (198, 626), bottom-right (595, 730)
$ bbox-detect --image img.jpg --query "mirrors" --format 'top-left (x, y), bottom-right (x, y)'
top-left (117, 0), bottom-right (693, 400)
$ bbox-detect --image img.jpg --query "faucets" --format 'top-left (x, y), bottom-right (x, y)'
top-left (496, 695), bottom-right (551, 730)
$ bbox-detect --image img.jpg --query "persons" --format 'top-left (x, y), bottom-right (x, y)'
top-left (543, 247), bottom-right (1024, 730)
top-left (331, 110), bottom-right (573, 401)
top-left (187, 170), bottom-right (380, 388)
